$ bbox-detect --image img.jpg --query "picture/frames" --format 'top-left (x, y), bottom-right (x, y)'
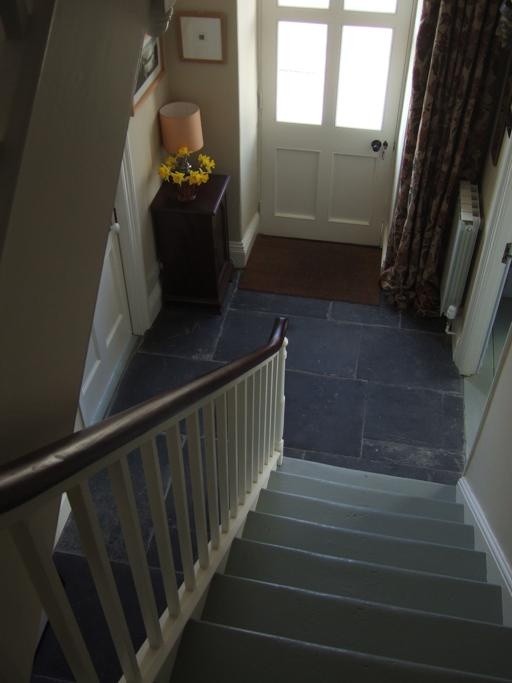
top-left (127, 30), bottom-right (168, 115)
top-left (174, 7), bottom-right (229, 64)
top-left (488, 63), bottom-right (511, 165)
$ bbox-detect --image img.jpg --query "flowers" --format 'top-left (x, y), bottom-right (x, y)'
top-left (159, 147), bottom-right (215, 187)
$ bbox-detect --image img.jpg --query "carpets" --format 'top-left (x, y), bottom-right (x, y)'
top-left (235, 231), bottom-right (383, 301)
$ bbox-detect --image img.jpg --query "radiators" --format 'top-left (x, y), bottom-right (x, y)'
top-left (439, 172), bottom-right (482, 338)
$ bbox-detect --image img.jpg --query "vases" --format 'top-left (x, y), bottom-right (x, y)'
top-left (174, 179), bottom-right (198, 201)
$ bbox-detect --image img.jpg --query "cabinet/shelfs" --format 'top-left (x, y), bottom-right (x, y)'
top-left (148, 172), bottom-right (236, 317)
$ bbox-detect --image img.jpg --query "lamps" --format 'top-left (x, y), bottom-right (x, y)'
top-left (160, 101), bottom-right (205, 171)
